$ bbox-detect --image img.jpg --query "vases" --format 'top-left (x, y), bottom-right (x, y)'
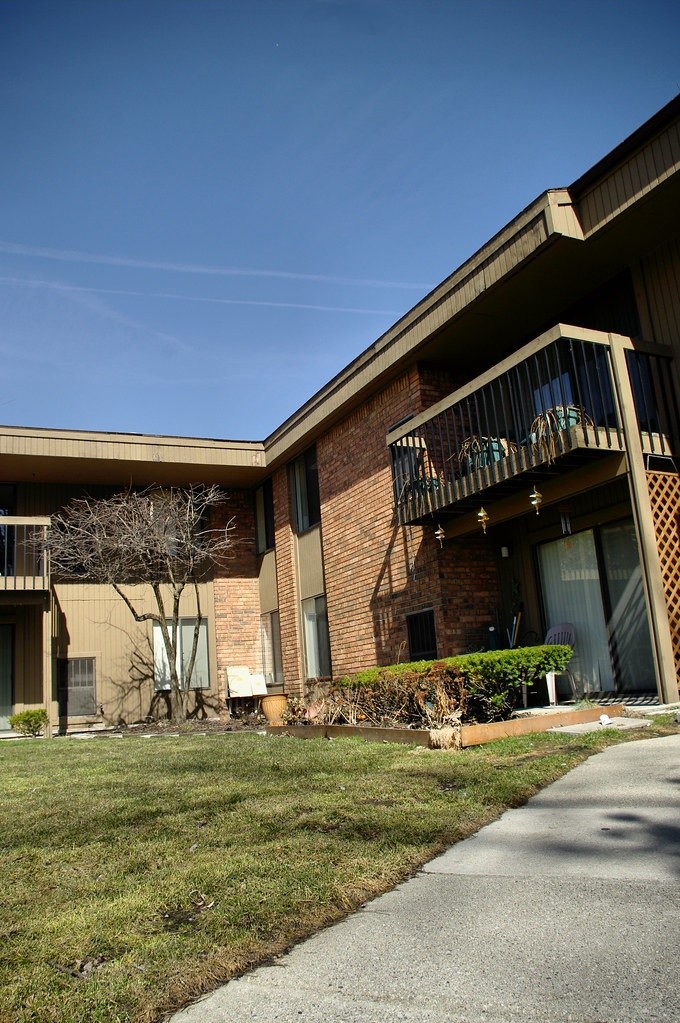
top-left (261, 693), bottom-right (289, 726)
top-left (545, 407), bottom-right (578, 433)
top-left (472, 441), bottom-right (500, 469)
top-left (415, 479), bottom-right (440, 493)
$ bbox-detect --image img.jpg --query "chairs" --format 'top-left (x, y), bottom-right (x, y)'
top-left (522, 622), bottom-right (579, 709)
